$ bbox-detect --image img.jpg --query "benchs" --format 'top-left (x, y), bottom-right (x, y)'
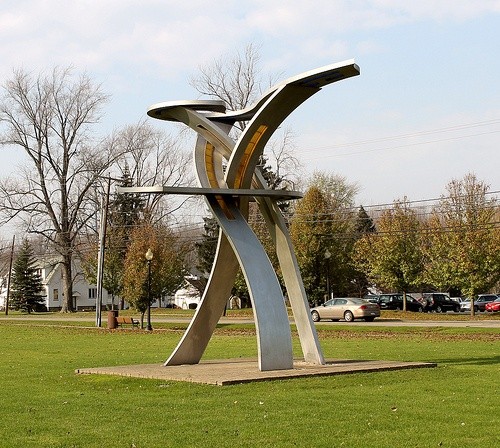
top-left (116, 316), bottom-right (139, 328)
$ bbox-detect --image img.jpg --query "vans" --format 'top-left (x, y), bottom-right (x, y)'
top-left (460, 294), bottom-right (498, 312)
top-left (379, 294), bottom-right (424, 313)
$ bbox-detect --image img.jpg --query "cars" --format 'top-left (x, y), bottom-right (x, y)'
top-left (309, 298), bottom-right (381, 322)
top-left (363, 294), bottom-right (379, 303)
top-left (445, 297), bottom-right (466, 304)
top-left (485, 297), bottom-right (500, 312)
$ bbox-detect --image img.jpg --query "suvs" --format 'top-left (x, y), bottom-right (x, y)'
top-left (421, 294), bottom-right (459, 313)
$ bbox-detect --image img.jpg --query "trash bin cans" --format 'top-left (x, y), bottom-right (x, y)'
top-left (108, 310), bottom-right (118, 329)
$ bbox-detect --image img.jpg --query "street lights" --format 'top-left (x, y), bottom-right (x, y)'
top-left (145, 248), bottom-right (154, 332)
